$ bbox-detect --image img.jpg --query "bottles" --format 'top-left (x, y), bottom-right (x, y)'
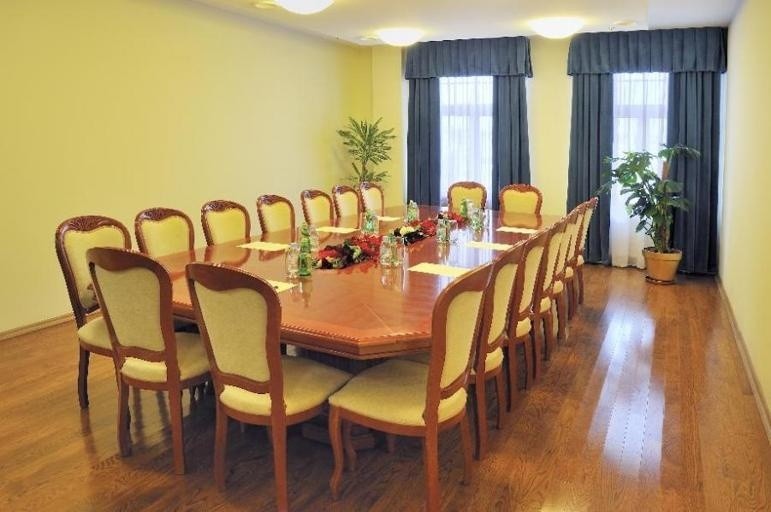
top-left (360, 198), bottom-right (487, 245)
top-left (394, 236), bottom-right (409, 269)
top-left (379, 235), bottom-right (392, 266)
top-left (308, 224), bottom-right (321, 252)
top-left (296, 221), bottom-right (312, 277)
top-left (386, 232), bottom-right (399, 267)
top-left (379, 266), bottom-right (395, 289)
top-left (287, 276), bottom-right (303, 304)
top-left (394, 262), bottom-right (410, 293)
top-left (298, 276), bottom-right (313, 309)
top-left (284, 242), bottom-right (300, 278)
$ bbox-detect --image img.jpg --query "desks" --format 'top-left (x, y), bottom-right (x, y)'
top-left (153, 203), bottom-right (564, 450)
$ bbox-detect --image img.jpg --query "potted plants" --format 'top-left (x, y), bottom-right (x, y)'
top-left (591, 143), bottom-right (705, 284)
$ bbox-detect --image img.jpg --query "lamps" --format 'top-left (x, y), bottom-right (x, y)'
top-left (529, 14), bottom-right (592, 43)
top-left (374, 28), bottom-right (431, 47)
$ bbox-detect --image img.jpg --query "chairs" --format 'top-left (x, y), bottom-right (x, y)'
top-left (184, 263), bottom-right (352, 509)
top-left (383, 249), bottom-right (385, 259)
top-left (84, 247), bottom-right (218, 475)
top-left (365, 180), bottom-right (601, 460)
top-left (134, 183), bottom-right (382, 246)
top-left (55, 215), bottom-right (198, 409)
top-left (328, 262), bottom-right (494, 508)
